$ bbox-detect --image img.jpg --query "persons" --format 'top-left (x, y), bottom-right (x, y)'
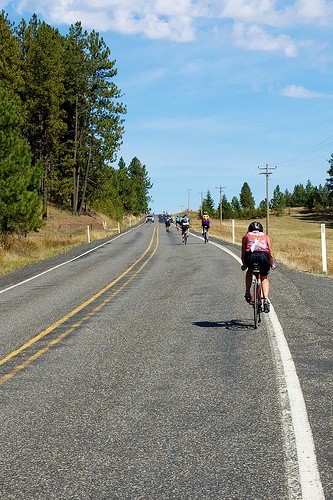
top-left (161, 211), bottom-right (211, 243)
top-left (242, 221), bottom-right (275, 314)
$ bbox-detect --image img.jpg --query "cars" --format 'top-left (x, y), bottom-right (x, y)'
top-left (145, 215), bottom-right (155, 223)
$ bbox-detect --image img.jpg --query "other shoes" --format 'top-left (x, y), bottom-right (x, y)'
top-left (246, 293), bottom-right (251, 303)
top-left (263, 298), bottom-right (270, 313)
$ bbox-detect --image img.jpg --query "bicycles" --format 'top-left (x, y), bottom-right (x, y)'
top-left (181, 227), bottom-right (188, 245)
top-left (202, 224), bottom-right (210, 244)
top-left (244, 263), bottom-right (268, 329)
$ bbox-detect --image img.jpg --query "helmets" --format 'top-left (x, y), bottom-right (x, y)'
top-left (248, 221), bottom-right (264, 232)
